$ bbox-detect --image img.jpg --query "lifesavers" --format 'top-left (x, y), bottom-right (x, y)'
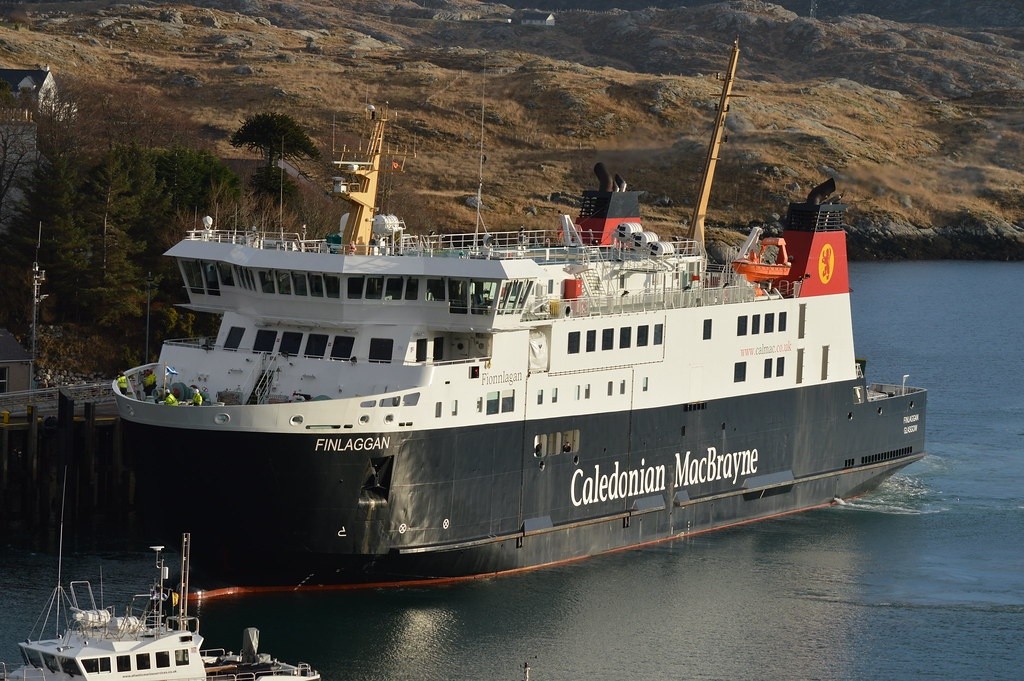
top-left (42, 415), bottom-right (58, 437)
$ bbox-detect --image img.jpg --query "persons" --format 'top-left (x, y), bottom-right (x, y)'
top-left (367, 105), bottom-right (375, 120)
top-left (115, 371), bottom-right (127, 396)
top-left (253, 238), bottom-right (259, 249)
top-left (350, 241), bottom-right (356, 255)
top-left (293, 237), bottom-right (298, 251)
top-left (186, 389), bottom-right (202, 407)
top-left (144, 369), bottom-right (157, 396)
top-left (164, 389), bottom-right (178, 406)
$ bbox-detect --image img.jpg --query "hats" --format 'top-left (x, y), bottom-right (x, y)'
top-left (164, 388), bottom-right (171, 394)
top-left (193, 388), bottom-right (199, 394)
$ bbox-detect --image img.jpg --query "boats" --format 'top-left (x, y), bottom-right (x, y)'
top-left (113, 34), bottom-right (929, 587)
top-left (0, 532), bottom-right (322, 681)
